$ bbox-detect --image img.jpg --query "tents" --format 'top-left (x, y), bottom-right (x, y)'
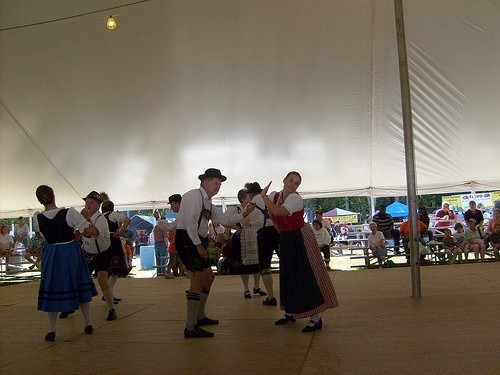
top-left (322, 208), bottom-right (362, 224)
top-left (127, 214), bottom-right (157, 232)
top-left (164, 210), bottom-right (177, 222)
top-left (369, 201), bottom-right (411, 220)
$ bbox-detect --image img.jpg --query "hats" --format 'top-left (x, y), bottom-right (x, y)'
top-left (245, 182), bottom-right (263, 194)
top-left (82, 191), bottom-right (108, 204)
top-left (167, 194), bottom-right (181, 204)
top-left (198, 168), bottom-right (226, 181)
top-left (468, 218), bottom-right (476, 224)
top-left (316, 209), bottom-right (324, 215)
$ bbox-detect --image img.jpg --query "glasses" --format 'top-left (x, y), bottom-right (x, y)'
top-left (469, 221), bottom-right (473, 224)
top-left (470, 203), bottom-right (474, 205)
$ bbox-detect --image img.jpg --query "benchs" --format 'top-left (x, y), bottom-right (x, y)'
top-left (349, 234), bottom-right (494, 269)
top-left (152, 245), bottom-right (330, 276)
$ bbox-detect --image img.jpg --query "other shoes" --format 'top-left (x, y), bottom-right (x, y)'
top-left (6, 266), bottom-right (10, 270)
top-left (253, 287), bottom-right (266, 295)
top-left (165, 270), bottom-right (175, 279)
top-left (29, 265), bottom-right (34, 270)
top-left (275, 313), bottom-right (296, 325)
top-left (102, 296), bottom-right (121, 304)
top-left (184, 324), bottom-right (214, 338)
top-left (60, 310), bottom-right (74, 318)
top-left (395, 250), bottom-right (401, 255)
top-left (263, 297), bottom-right (277, 306)
top-left (45, 332), bottom-right (56, 342)
top-left (244, 290), bottom-right (251, 298)
top-left (197, 317), bottom-right (219, 325)
top-left (107, 308), bottom-right (116, 321)
top-left (85, 324), bottom-right (93, 335)
top-left (302, 317), bottom-right (322, 333)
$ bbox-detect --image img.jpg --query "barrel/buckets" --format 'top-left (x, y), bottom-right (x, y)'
top-left (140, 245), bottom-right (155, 271)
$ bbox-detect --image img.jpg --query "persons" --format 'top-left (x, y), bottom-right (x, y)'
top-left (58, 191), bottom-right (117, 321)
top-left (0, 211), bottom-right (46, 271)
top-left (260, 171), bottom-right (338, 332)
top-left (32, 184), bottom-right (99, 340)
top-left (175, 168), bottom-right (255, 340)
top-left (311, 201), bottom-right (500, 268)
top-left (208, 182), bottom-right (280, 306)
top-left (101, 193), bottom-right (192, 304)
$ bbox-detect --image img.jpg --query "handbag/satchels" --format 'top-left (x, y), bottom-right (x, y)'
top-left (407, 241), bottom-right (426, 255)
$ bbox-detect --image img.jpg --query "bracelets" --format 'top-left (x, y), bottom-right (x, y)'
top-left (262, 195), bottom-right (268, 198)
